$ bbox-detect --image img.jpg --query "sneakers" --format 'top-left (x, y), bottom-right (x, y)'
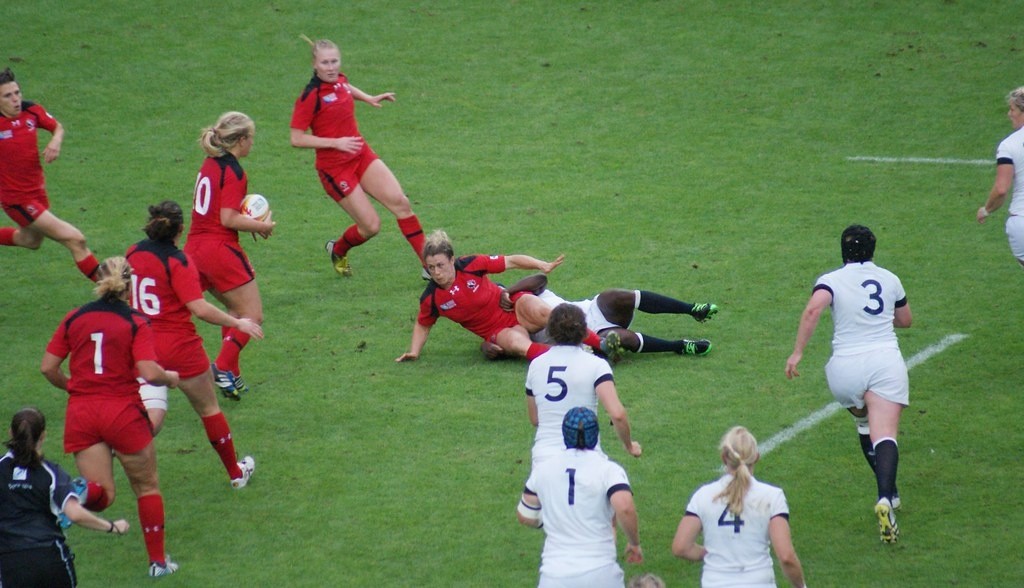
top-left (209, 364), bottom-right (241, 401)
top-left (55, 477), bottom-right (88, 529)
top-left (891, 493), bottom-right (904, 513)
top-left (679, 338), bottom-right (712, 358)
top-left (325, 240), bottom-right (353, 277)
top-left (600, 331), bottom-right (623, 364)
top-left (149, 554), bottom-right (180, 578)
top-left (229, 455), bottom-right (256, 490)
top-left (421, 266), bottom-right (435, 282)
top-left (689, 301), bottom-right (720, 324)
top-left (228, 369), bottom-right (250, 394)
top-left (873, 497), bottom-right (900, 546)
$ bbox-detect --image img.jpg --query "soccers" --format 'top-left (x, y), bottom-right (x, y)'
top-left (239, 194), bottom-right (269, 222)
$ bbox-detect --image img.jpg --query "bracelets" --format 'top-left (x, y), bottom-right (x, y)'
top-left (107, 519), bottom-right (113, 533)
top-left (983, 206), bottom-right (991, 217)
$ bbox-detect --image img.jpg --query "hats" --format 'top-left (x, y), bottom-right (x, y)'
top-left (562, 407), bottom-right (599, 449)
top-left (841, 225), bottom-right (876, 260)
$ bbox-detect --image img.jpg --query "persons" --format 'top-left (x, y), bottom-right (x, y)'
top-left (41, 256), bottom-right (179, 576)
top-left (525, 303), bottom-right (642, 470)
top-left (392, 229), bottom-right (625, 364)
top-left (126, 200), bottom-right (255, 489)
top-left (626, 573), bottom-right (666, 588)
top-left (673, 426), bottom-right (806, 588)
top-left (0, 66), bottom-right (100, 283)
top-left (516, 406), bottom-right (644, 588)
top-left (784, 225), bottom-right (913, 544)
top-left (0, 407), bottom-right (130, 588)
top-left (290, 34), bottom-right (431, 280)
top-left (978, 88), bottom-right (1024, 267)
top-left (480, 273), bottom-right (717, 357)
top-left (183, 110), bottom-right (277, 399)
